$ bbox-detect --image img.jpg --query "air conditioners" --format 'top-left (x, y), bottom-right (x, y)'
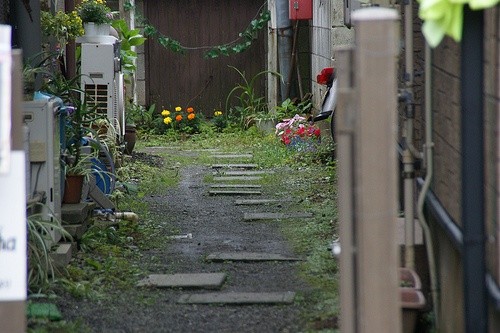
top-left (78, 44), bottom-right (127, 145)
top-left (24, 95), bottom-right (64, 246)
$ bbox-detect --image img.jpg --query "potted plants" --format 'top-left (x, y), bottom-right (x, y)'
top-left (74, 0), bottom-right (112, 35)
top-left (62, 100), bottom-right (105, 205)
top-left (400, 267), bottom-right (423, 290)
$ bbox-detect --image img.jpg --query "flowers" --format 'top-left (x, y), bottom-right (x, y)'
top-left (275, 114), bottom-right (321, 151)
top-left (161, 105), bottom-right (225, 131)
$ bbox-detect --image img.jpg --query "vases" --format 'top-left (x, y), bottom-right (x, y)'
top-left (399, 288), bottom-right (427, 333)
